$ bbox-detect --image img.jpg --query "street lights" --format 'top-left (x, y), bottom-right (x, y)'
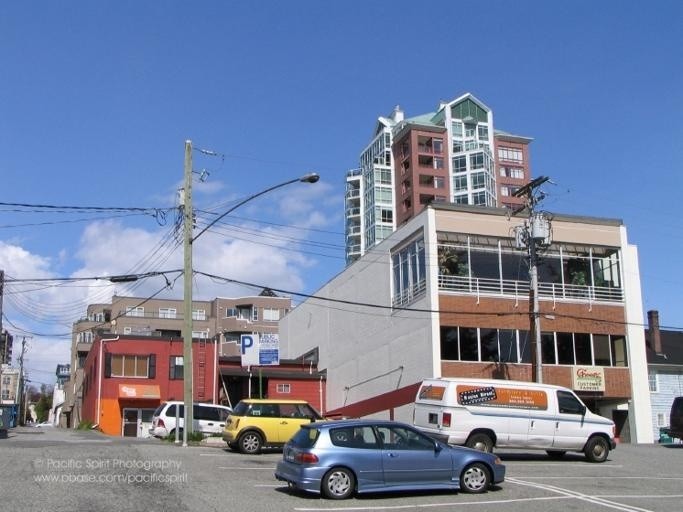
top-left (183, 139), bottom-right (320, 435)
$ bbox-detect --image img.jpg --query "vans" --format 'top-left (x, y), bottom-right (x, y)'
top-left (660, 396), bottom-right (683, 440)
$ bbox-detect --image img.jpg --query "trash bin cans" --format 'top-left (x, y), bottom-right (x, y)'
top-left (660, 427), bottom-right (672, 444)
